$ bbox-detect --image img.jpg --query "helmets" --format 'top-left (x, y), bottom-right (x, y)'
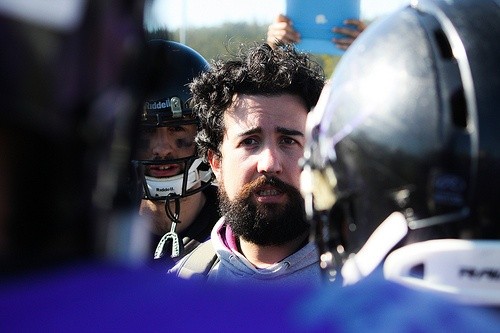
top-left (297, 0), bottom-right (500, 307)
top-left (122, 37), bottom-right (216, 199)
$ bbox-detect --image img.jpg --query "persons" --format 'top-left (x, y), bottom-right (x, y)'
top-left (165, 40), bottom-right (325, 286)
top-left (298, 0), bottom-right (500, 313)
top-left (266, 11), bottom-right (367, 53)
top-left (0, 0), bottom-right (500, 332)
top-left (132, 35), bottom-right (227, 276)
